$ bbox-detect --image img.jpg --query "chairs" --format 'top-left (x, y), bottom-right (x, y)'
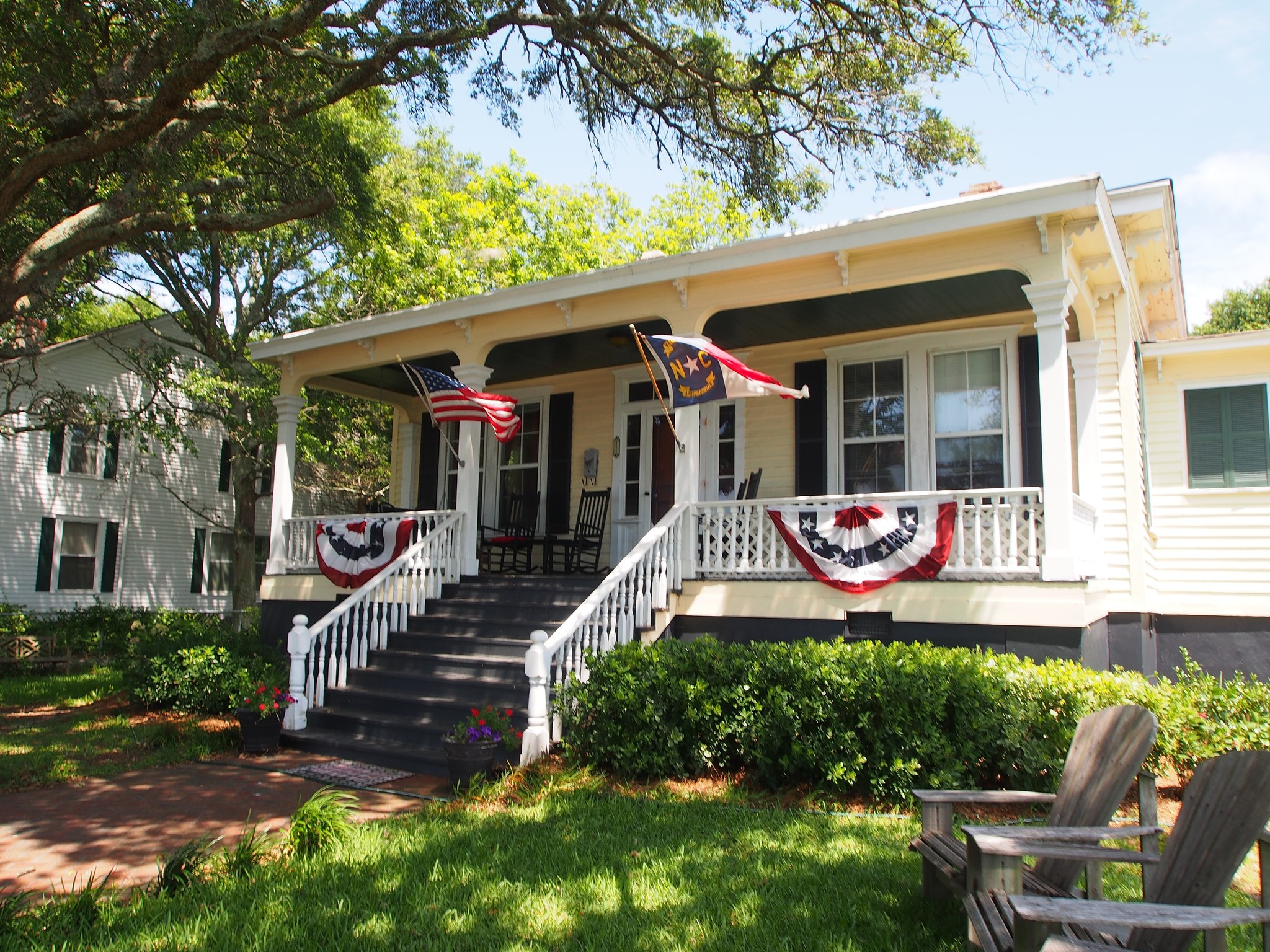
top-left (698, 466), bottom-right (764, 578)
top-left (960, 749), bottom-right (1270, 952)
top-left (479, 491), bottom-right (542, 574)
top-left (906, 705), bottom-right (1166, 903)
top-left (540, 485), bottom-right (612, 575)
top-left (379, 502), bottom-right (395, 513)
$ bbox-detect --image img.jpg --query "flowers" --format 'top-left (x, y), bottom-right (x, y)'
top-left (238, 678), bottom-right (300, 725)
top-left (445, 701), bottom-right (522, 753)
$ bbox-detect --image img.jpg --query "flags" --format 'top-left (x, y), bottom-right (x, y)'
top-left (637, 330), bottom-right (811, 409)
top-left (402, 359), bottom-right (522, 444)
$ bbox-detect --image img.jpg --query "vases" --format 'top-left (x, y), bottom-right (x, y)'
top-left (442, 734), bottom-right (502, 791)
top-left (237, 708), bottom-right (286, 752)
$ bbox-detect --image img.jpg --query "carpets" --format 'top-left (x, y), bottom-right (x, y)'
top-left (286, 758), bottom-right (416, 790)
